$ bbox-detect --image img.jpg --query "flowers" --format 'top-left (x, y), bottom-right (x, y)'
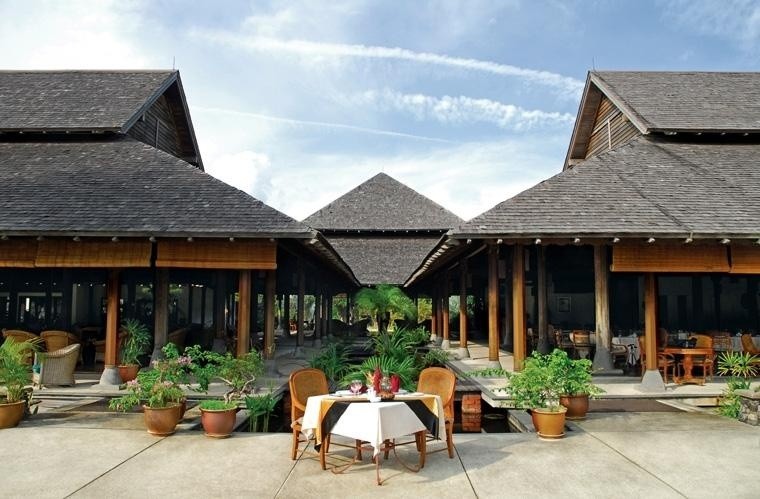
top-left (107, 355), bottom-right (190, 412)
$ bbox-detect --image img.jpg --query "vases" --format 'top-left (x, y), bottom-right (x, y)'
top-left (142, 402), bottom-right (181, 435)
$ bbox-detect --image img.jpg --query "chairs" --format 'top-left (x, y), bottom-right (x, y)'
top-left (0, 321), bottom-right (192, 387)
top-left (546, 324), bottom-right (760, 384)
top-left (383, 367), bottom-right (456, 470)
top-left (288, 368), bottom-right (363, 470)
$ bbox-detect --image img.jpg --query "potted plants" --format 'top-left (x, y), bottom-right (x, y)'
top-left (559, 358), bottom-right (592, 420)
top-left (460, 349), bottom-right (606, 439)
top-left (189, 345), bottom-right (273, 437)
top-left (0, 334), bottom-right (40, 428)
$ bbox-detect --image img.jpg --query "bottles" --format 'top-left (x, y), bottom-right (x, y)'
top-left (378, 378), bottom-right (392, 394)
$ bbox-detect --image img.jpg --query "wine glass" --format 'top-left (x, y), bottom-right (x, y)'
top-left (351, 380), bottom-right (362, 395)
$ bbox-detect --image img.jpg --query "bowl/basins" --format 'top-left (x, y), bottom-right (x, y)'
top-left (376, 393), bottom-right (395, 400)
